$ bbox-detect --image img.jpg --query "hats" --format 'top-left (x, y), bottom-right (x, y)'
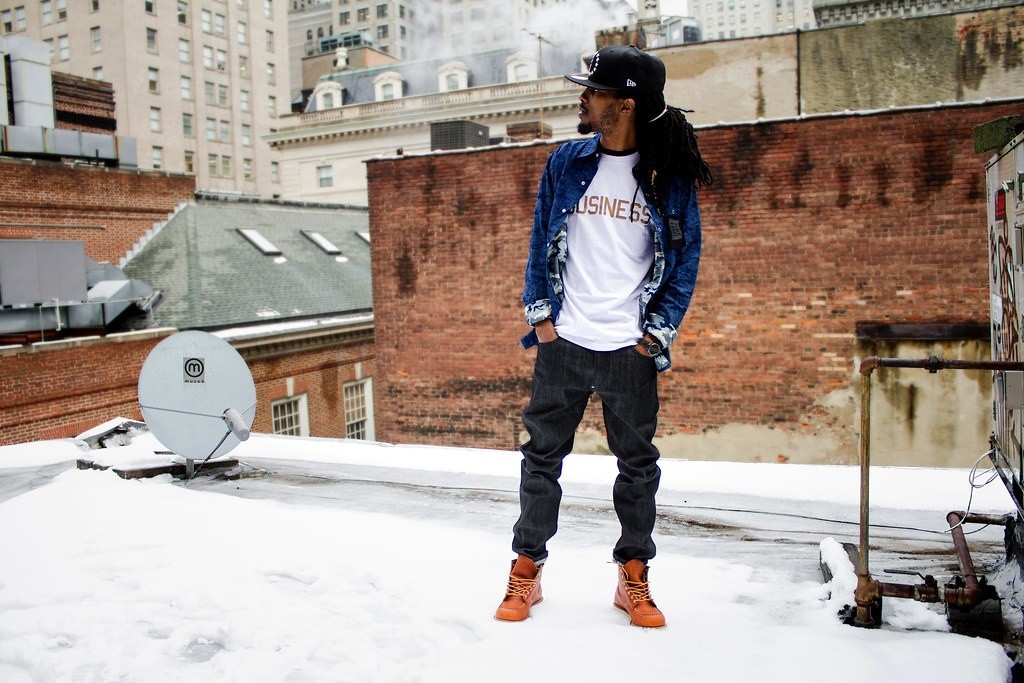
top-left (564, 44), bottom-right (666, 101)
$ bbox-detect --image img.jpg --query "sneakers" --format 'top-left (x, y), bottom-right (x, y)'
top-left (496, 555), bottom-right (544, 621)
top-left (613, 559), bottom-right (665, 627)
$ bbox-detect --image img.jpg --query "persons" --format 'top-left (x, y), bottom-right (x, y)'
top-left (498, 45), bottom-right (709, 627)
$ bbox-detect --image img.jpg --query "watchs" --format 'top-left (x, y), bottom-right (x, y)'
top-left (637, 337), bottom-right (661, 357)
